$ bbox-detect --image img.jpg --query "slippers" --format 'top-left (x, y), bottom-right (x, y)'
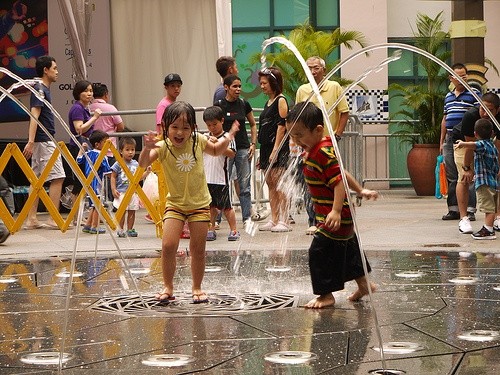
top-left (228, 231), bottom-right (240, 240)
top-left (271, 222), bottom-right (292, 232)
top-left (191, 293), bottom-right (208, 303)
top-left (259, 221), bottom-right (277, 231)
top-left (127, 228), bottom-right (137, 236)
top-left (46, 222), bottom-right (73, 229)
top-left (117, 229), bottom-right (125, 237)
top-left (206, 230), bottom-right (217, 240)
top-left (157, 292), bottom-right (175, 300)
top-left (23, 222), bottom-right (44, 229)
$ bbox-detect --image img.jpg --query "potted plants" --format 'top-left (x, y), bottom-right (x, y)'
top-left (383, 10), bottom-right (451, 196)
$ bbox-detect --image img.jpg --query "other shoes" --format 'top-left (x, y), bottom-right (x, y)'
top-left (442, 211), bottom-right (458, 219)
top-left (214, 221), bottom-right (219, 230)
top-left (306, 226), bottom-right (317, 235)
top-left (467, 212), bottom-right (475, 220)
top-left (145, 213), bottom-right (154, 223)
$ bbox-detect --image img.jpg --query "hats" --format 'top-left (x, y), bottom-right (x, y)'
top-left (163, 74), bottom-right (182, 86)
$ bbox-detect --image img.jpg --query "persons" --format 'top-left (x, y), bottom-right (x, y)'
top-left (200, 106), bottom-right (239, 240)
top-left (257, 67), bottom-right (293, 233)
top-left (452, 92), bottom-right (500, 234)
top-left (111, 135), bottom-right (144, 237)
top-left (139, 102), bottom-right (241, 303)
top-left (69, 80), bottom-right (102, 225)
top-left (214, 56), bottom-right (239, 101)
top-left (440, 62), bottom-right (483, 222)
top-left (23, 56), bottom-right (75, 230)
top-left (76, 130), bottom-right (112, 234)
top-left (294, 56), bottom-right (350, 235)
top-left (286, 101), bottom-right (379, 310)
top-left (86, 83), bottom-right (126, 208)
top-left (454, 119), bottom-right (499, 240)
top-left (145, 74), bottom-right (183, 225)
top-left (214, 74), bottom-right (257, 230)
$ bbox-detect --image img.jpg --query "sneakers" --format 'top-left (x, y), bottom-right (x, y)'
top-left (493, 216), bottom-right (500, 230)
top-left (458, 215), bottom-right (472, 234)
top-left (471, 225), bottom-right (496, 240)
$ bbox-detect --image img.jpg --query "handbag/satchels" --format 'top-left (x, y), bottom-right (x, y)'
top-left (435, 154), bottom-right (448, 200)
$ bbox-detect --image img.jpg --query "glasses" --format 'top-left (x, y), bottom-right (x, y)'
top-left (261, 67), bottom-right (277, 81)
top-left (451, 74), bottom-right (468, 80)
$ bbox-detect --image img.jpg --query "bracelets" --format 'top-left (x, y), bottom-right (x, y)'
top-left (143, 147), bottom-right (150, 154)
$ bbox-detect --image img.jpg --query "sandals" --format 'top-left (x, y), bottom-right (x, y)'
top-left (82, 225), bottom-right (90, 232)
top-left (90, 227), bottom-right (105, 234)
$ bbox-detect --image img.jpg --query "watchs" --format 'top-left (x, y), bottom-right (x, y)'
top-left (462, 166), bottom-right (470, 171)
top-left (335, 134), bottom-right (341, 140)
top-left (249, 142), bottom-right (256, 146)
